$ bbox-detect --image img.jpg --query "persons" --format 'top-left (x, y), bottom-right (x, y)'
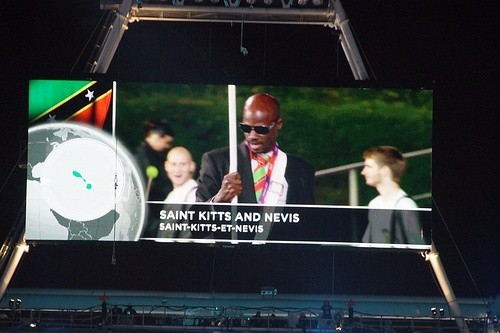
top-left (156, 147), bottom-right (212, 239)
top-left (130, 118), bottom-right (177, 238)
top-left (361, 145), bottom-right (423, 244)
top-left (187, 92), bottom-right (315, 239)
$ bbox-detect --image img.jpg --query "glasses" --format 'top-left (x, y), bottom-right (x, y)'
top-left (240, 118), bottom-right (279, 135)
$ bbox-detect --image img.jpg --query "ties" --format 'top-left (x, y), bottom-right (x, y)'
top-left (252, 154), bottom-right (270, 203)
